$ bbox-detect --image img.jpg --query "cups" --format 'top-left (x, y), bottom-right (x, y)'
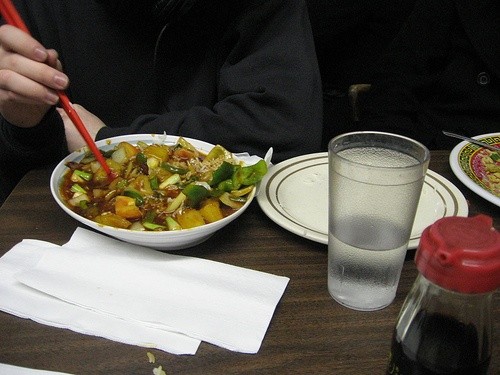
top-left (328, 130), bottom-right (431, 312)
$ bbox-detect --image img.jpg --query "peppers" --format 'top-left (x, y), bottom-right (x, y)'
top-left (180, 160), bottom-right (268, 210)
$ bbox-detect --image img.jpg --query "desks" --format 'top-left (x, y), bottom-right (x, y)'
top-left (0, 149), bottom-right (500, 375)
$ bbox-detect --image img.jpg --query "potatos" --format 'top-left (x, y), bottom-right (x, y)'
top-left (91, 141), bottom-right (224, 233)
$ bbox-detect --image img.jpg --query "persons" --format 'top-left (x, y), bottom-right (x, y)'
top-left (0, 0), bottom-right (326, 201)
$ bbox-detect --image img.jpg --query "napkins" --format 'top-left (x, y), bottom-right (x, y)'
top-left (18, 227), bottom-right (291, 354)
top-left (0, 239), bottom-right (202, 356)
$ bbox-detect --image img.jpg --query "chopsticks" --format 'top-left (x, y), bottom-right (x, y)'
top-left (0, 0), bottom-right (112, 176)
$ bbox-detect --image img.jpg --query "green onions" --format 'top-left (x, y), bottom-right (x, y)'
top-left (71, 143), bottom-right (186, 231)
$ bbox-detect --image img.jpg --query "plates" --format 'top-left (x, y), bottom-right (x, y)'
top-left (257, 152), bottom-right (468, 251)
top-left (449, 132), bottom-right (500, 208)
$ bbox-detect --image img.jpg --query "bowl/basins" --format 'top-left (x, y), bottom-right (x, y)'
top-left (50, 134), bottom-right (256, 249)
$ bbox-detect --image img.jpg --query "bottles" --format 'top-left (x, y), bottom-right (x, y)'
top-left (386, 213), bottom-right (500, 375)
